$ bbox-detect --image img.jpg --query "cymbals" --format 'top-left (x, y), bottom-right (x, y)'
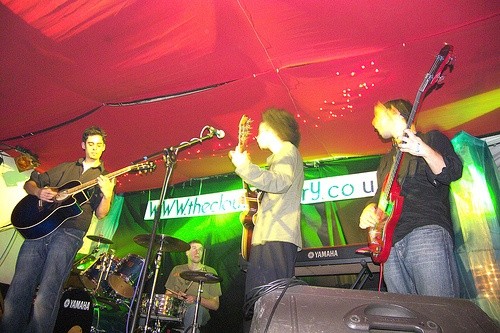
top-left (180, 271), bottom-right (223, 284)
top-left (86, 236), bottom-right (113, 245)
top-left (134, 234), bottom-right (191, 252)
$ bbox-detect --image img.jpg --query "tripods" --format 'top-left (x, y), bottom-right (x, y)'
top-left (182, 279), bottom-right (203, 333)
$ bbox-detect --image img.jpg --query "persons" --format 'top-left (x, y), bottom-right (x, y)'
top-left (53, 273), bottom-right (94, 333)
top-left (165, 240), bottom-right (222, 333)
top-left (359, 98), bottom-right (463, 298)
top-left (227, 109), bottom-right (306, 310)
top-left (0, 127), bottom-right (115, 333)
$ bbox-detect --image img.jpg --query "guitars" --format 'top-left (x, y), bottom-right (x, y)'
top-left (235, 114), bottom-right (258, 261)
top-left (11, 161), bottom-right (157, 241)
top-left (356, 45), bottom-right (455, 265)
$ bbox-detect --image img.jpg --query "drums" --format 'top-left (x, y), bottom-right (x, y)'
top-left (65, 252), bottom-right (97, 289)
top-left (52, 287), bottom-right (139, 333)
top-left (81, 252), bottom-right (124, 292)
top-left (108, 254), bottom-right (154, 298)
top-left (139, 293), bottom-right (185, 321)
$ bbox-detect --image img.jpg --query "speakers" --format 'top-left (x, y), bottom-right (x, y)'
top-left (49, 286), bottom-right (94, 333)
top-left (247, 285), bottom-right (500, 333)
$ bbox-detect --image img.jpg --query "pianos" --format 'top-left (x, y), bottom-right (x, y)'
top-left (294, 242), bottom-right (383, 290)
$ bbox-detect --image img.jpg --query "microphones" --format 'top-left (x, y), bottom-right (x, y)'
top-left (206, 126), bottom-right (226, 139)
